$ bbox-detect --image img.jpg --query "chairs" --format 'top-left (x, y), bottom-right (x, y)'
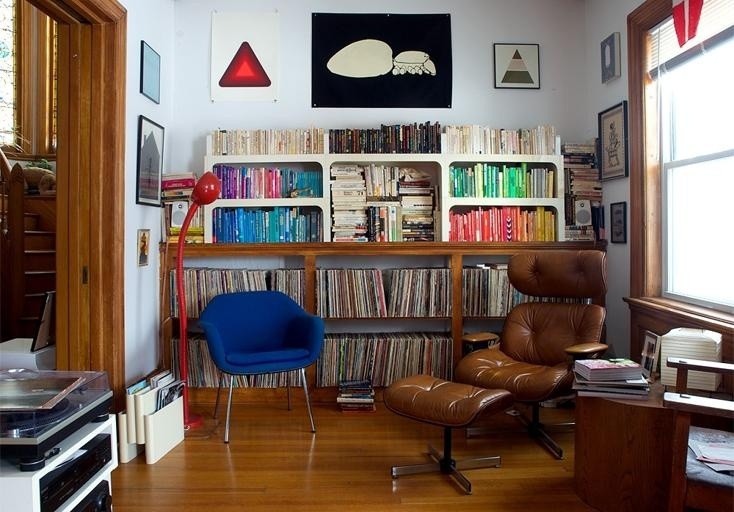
top-left (457, 250), bottom-right (608, 461)
top-left (662, 354), bottom-right (734, 511)
top-left (196, 291), bottom-right (323, 443)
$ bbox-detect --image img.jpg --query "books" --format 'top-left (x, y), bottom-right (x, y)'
top-left (126, 380), bottom-right (148, 396)
top-left (328, 120), bottom-right (442, 153)
top-left (161, 173), bottom-right (204, 244)
top-left (571, 358), bottom-right (651, 401)
top-left (168, 266), bottom-right (305, 389)
top-left (315, 267), bottom-right (453, 387)
top-left (448, 205), bottom-right (556, 243)
top-left (449, 162), bottom-right (554, 198)
top-left (212, 164), bottom-right (323, 198)
top-left (150, 370), bottom-right (185, 409)
top-left (562, 144), bottom-right (603, 240)
top-left (461, 265), bottom-right (583, 317)
top-left (330, 163), bottom-right (442, 242)
top-left (337, 380), bottom-right (376, 414)
top-left (210, 126), bottom-right (325, 154)
top-left (212, 207), bottom-right (323, 244)
top-left (444, 124), bottom-right (561, 154)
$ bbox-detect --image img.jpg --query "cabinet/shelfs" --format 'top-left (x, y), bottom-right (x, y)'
top-left (203, 135), bottom-right (567, 242)
top-left (574, 376), bottom-right (677, 512)
top-left (159, 239), bottom-right (608, 404)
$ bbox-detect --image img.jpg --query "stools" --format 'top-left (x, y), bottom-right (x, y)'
top-left (383, 373), bottom-right (511, 494)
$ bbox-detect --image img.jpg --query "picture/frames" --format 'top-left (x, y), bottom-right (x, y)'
top-left (493, 42), bottom-right (541, 90)
top-left (609, 202), bottom-right (626, 244)
top-left (598, 100), bottom-right (628, 182)
top-left (31, 290), bottom-right (57, 352)
top-left (136, 228), bottom-right (150, 267)
top-left (136, 115), bottom-right (165, 208)
top-left (140, 39), bottom-right (161, 104)
